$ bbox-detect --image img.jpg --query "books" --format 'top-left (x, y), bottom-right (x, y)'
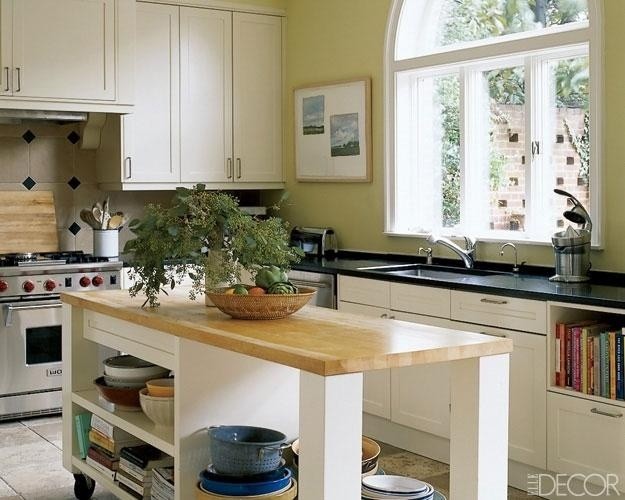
top-left (71, 412), bottom-right (174, 500)
top-left (553, 315), bottom-right (625, 402)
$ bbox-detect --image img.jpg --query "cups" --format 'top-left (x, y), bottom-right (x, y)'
top-left (92, 230), bottom-right (119, 258)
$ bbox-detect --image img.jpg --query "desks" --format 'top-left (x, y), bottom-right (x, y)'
top-left (61, 287), bottom-right (515, 500)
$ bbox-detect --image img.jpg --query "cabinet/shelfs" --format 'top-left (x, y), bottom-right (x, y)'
top-left (119, 261), bottom-right (207, 291)
top-left (450, 283), bottom-right (546, 499)
top-left (547, 298), bottom-right (625, 500)
top-left (0, 0), bottom-right (136, 120)
top-left (94, 0), bottom-right (178, 191)
top-left (181, 0), bottom-right (285, 191)
top-left (336, 271), bottom-right (450, 467)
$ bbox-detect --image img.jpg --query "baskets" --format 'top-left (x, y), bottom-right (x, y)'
top-left (204, 285), bottom-right (318, 320)
top-left (208, 423), bottom-right (288, 474)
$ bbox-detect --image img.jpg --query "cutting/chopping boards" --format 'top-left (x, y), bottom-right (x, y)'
top-left (0, 190), bottom-right (61, 254)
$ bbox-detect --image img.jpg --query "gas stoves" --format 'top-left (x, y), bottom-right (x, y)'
top-left (0, 249), bottom-right (123, 301)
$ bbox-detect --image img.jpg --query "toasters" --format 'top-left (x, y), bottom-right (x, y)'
top-left (287, 224), bottom-right (338, 257)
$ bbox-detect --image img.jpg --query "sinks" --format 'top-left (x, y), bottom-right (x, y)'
top-left (356, 263), bottom-right (498, 280)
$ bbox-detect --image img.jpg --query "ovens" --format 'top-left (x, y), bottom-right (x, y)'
top-left (0, 299), bottom-right (64, 422)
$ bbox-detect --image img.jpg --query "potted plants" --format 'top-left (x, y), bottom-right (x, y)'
top-left (124, 182), bottom-right (305, 307)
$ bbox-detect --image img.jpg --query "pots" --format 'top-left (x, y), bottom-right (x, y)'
top-left (202, 425), bottom-right (298, 476)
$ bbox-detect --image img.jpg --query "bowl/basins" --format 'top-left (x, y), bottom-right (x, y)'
top-left (361, 474), bottom-right (447, 500)
top-left (193, 459), bottom-right (298, 500)
top-left (93, 354), bottom-right (173, 427)
top-left (293, 459), bottom-right (378, 484)
top-left (291, 435), bottom-right (380, 473)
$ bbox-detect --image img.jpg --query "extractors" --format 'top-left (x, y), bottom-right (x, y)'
top-left (0, 108), bottom-right (96, 128)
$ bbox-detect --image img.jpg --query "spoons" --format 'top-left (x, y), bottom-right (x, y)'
top-left (92, 208), bottom-right (102, 224)
top-left (109, 216), bottom-right (121, 230)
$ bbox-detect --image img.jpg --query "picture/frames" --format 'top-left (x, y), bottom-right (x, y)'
top-left (290, 75), bottom-right (373, 182)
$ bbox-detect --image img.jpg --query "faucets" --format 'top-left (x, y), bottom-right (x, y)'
top-left (418, 247), bottom-right (432, 265)
top-left (426, 234), bottom-right (476, 270)
top-left (500, 243), bottom-right (524, 273)
